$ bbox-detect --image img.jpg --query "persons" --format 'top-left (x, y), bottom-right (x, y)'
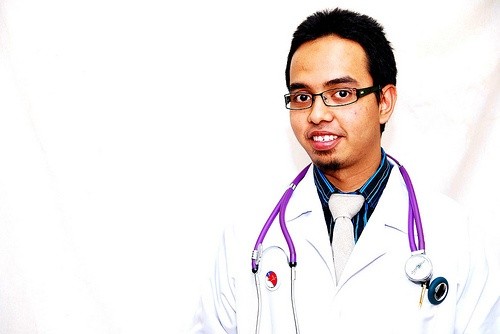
top-left (199, 8), bottom-right (481, 334)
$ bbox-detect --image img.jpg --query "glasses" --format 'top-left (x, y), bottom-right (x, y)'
top-left (284, 85), bottom-right (378, 109)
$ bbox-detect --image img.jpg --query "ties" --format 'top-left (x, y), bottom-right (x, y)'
top-left (328, 192), bottom-right (364, 282)
